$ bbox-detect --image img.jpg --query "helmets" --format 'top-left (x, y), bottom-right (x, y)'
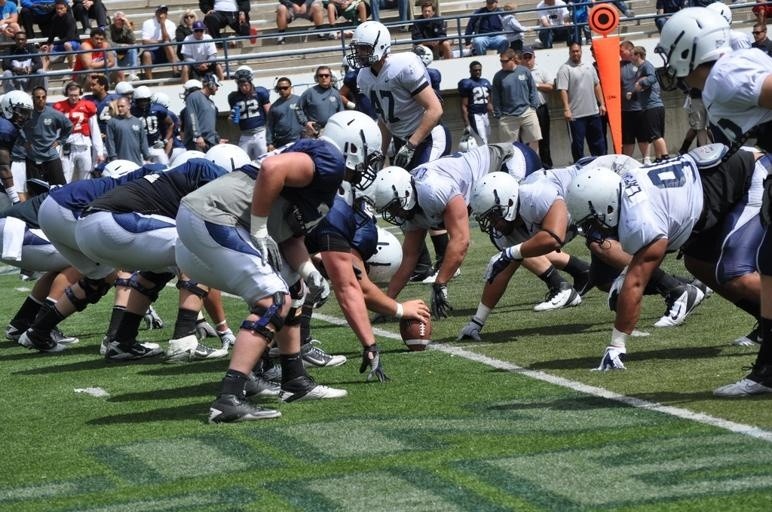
top-left (565, 166), bottom-right (624, 241)
top-left (470, 171), bottom-right (519, 239)
top-left (459, 136), bottom-right (477, 152)
top-left (654, 3), bottom-right (732, 91)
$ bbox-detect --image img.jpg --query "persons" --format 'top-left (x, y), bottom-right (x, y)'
top-left (347, 1), bottom-right (771, 395)
top-left (0, 0), bottom-right (432, 424)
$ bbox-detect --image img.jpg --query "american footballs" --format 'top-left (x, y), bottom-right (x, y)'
top-left (400, 302), bottom-right (432, 351)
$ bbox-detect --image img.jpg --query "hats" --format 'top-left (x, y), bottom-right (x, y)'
top-left (522, 47), bottom-right (533, 54)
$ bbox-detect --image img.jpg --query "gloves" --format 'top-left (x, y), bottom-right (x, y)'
top-left (359, 344), bottom-right (392, 383)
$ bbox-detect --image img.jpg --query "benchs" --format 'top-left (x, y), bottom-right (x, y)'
top-left (600, 1), bottom-right (771, 41)
top-left (311, 0), bottom-right (600, 76)
